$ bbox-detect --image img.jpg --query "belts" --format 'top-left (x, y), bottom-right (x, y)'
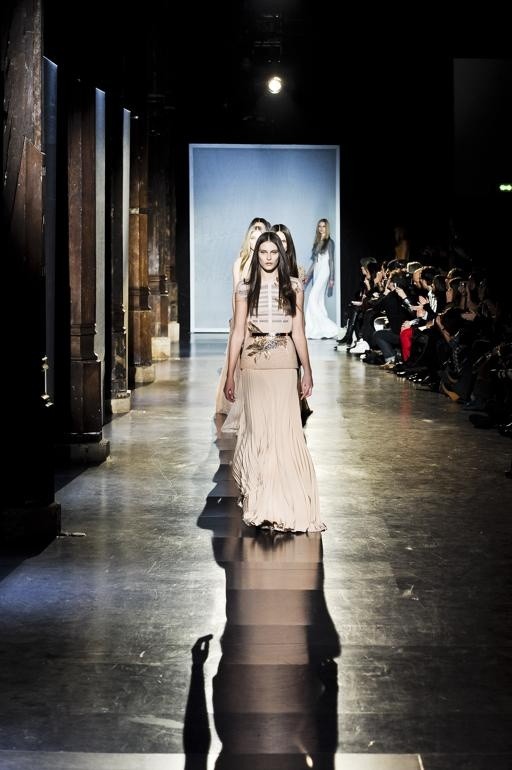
top-left (252, 333), bottom-right (290, 336)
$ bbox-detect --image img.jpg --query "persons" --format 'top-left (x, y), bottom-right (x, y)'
top-left (336, 228), bottom-right (512, 438)
top-left (214, 218), bottom-right (326, 535)
top-left (304, 219), bottom-right (334, 340)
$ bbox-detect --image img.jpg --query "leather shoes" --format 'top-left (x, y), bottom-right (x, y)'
top-left (334, 339), bottom-right (436, 392)
